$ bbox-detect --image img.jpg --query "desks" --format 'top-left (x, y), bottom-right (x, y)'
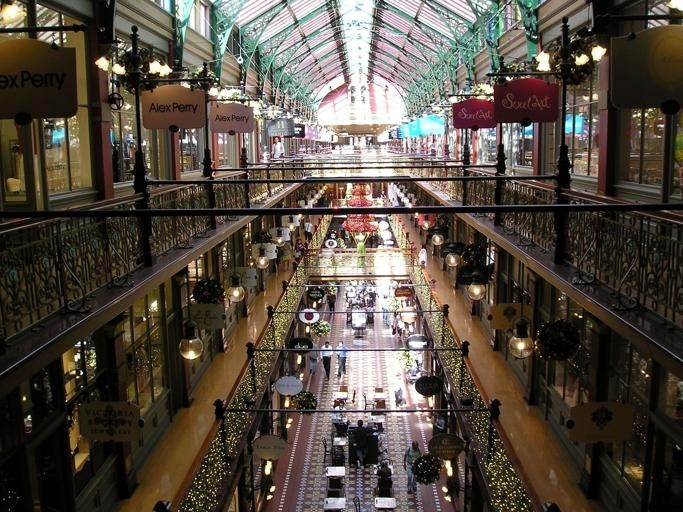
top-left (374, 497), bottom-right (397, 512)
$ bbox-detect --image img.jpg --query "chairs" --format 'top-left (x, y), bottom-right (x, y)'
top-left (322, 386), bottom-right (393, 512)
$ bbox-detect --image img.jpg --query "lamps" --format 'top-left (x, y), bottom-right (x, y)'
top-left (178, 192), bottom-right (297, 360)
top-left (94, 47), bottom-right (175, 78)
top-left (533, 38), bottom-right (609, 72)
top-left (422, 192), bottom-right (535, 359)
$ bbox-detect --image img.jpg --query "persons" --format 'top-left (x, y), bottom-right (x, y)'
top-left (403, 440), bottom-right (422, 494)
top-left (335, 340), bottom-right (349, 378)
top-left (334, 401), bottom-right (346, 414)
top-left (319, 341), bottom-right (333, 381)
top-left (270, 136), bottom-right (285, 162)
top-left (326, 293), bottom-right (337, 316)
top-left (376, 461), bottom-right (392, 477)
top-left (351, 420), bottom-right (370, 470)
top-left (343, 279), bottom-right (391, 328)
top-left (282, 218), bottom-right (314, 272)
top-left (391, 372), bottom-right (408, 408)
top-left (309, 338), bottom-right (319, 376)
top-left (410, 360), bottom-right (418, 376)
